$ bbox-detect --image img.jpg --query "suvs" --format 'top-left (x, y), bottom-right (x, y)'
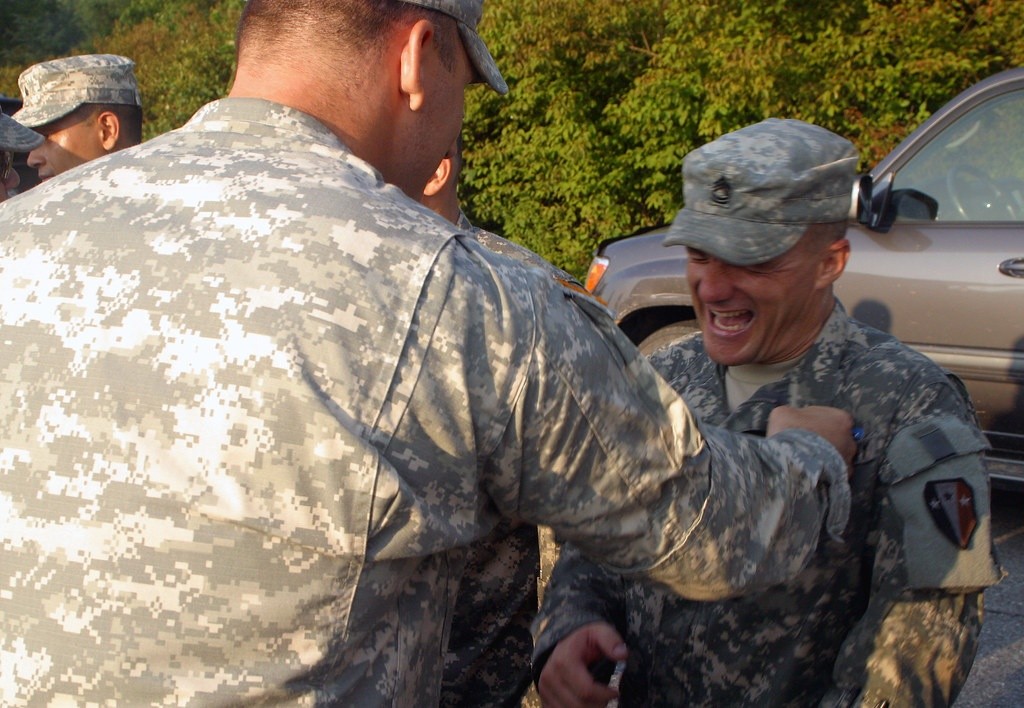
top-left (583, 66), bottom-right (1023, 490)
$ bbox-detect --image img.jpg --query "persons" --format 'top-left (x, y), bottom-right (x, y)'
top-left (529, 116), bottom-right (1007, 708)
top-left (0, 0), bottom-right (859, 708)
top-left (0, 108), bottom-right (45, 202)
top-left (10, 55), bottom-right (145, 182)
top-left (417, 132), bottom-right (477, 231)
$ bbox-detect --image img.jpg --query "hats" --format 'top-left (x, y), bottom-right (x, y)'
top-left (12, 53), bottom-right (141, 129)
top-left (0, 108), bottom-right (45, 152)
top-left (661, 118), bottom-right (858, 266)
top-left (400, 0), bottom-right (508, 95)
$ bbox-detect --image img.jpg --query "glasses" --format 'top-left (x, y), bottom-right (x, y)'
top-left (0, 155), bottom-right (14, 181)
